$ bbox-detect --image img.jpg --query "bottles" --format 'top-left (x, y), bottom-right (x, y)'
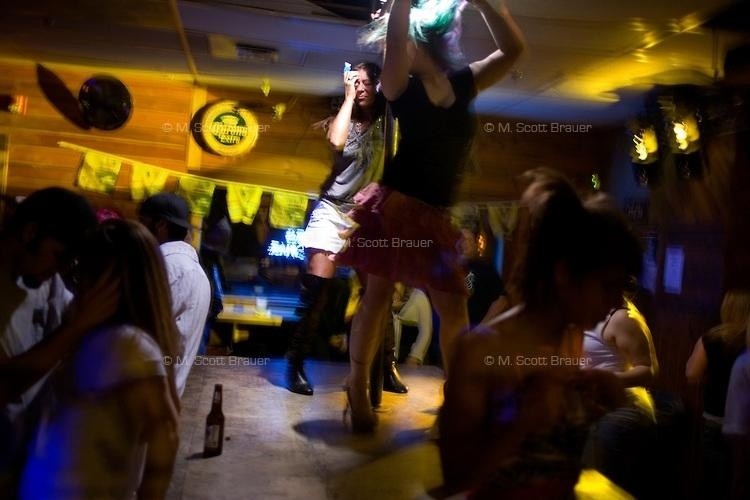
top-left (248, 191), bottom-right (273, 248)
top-left (200, 185), bottom-right (235, 263)
top-left (203, 384), bottom-right (226, 458)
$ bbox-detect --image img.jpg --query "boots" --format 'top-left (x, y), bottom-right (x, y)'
top-left (383, 310), bottom-right (408, 393)
top-left (285, 273), bottom-right (335, 394)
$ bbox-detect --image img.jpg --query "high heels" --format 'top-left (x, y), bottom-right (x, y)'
top-left (341, 365), bottom-right (379, 435)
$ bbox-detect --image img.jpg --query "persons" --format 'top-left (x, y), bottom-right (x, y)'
top-left (327, 0), bottom-right (526, 435)
top-left (284, 61), bottom-right (414, 396)
top-left (0, 168), bottom-right (750, 500)
